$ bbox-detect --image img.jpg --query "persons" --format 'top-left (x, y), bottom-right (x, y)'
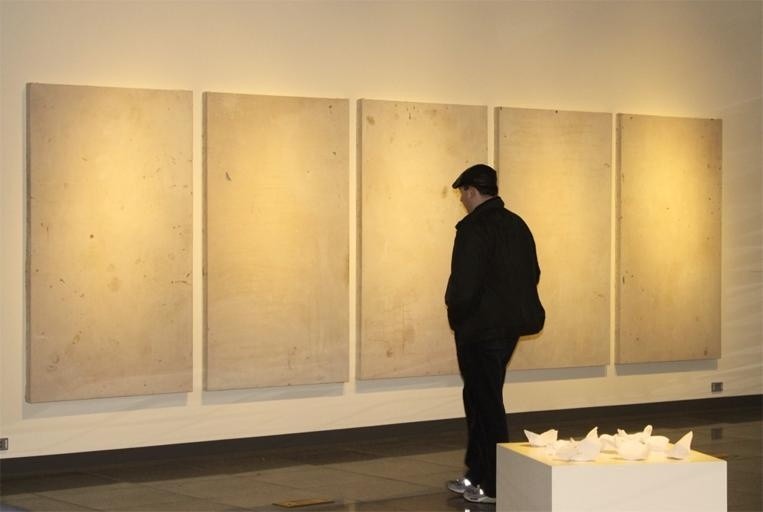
top-left (445, 164), bottom-right (546, 503)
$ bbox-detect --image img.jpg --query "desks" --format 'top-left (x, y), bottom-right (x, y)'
top-left (495, 442), bottom-right (728, 512)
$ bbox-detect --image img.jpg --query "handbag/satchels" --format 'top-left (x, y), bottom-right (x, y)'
top-left (522, 286), bottom-right (545, 335)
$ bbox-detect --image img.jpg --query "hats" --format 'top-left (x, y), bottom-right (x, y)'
top-left (452, 165), bottom-right (497, 188)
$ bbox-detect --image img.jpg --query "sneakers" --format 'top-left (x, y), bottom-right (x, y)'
top-left (447, 472), bottom-right (496, 503)
